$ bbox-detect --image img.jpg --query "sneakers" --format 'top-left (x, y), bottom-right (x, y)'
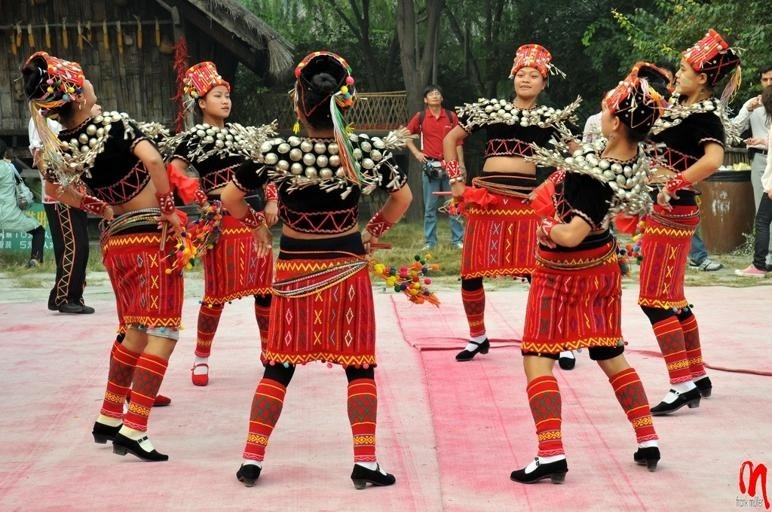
top-left (193, 361), bottom-right (208, 385)
top-left (31, 259), bottom-right (41, 268)
top-left (689, 259), bottom-right (723, 272)
top-left (734, 264), bottom-right (768, 278)
top-left (127, 389), bottom-right (171, 405)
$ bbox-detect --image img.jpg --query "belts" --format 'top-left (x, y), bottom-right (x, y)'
top-left (753, 148), bottom-right (768, 154)
top-left (425, 157), bottom-right (441, 161)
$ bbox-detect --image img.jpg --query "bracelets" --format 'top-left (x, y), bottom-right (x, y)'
top-left (262, 181), bottom-right (278, 201)
top-left (156, 192), bottom-right (175, 215)
top-left (539, 217), bottom-right (556, 234)
top-left (79, 193), bottom-right (109, 216)
top-left (548, 170), bottom-right (566, 186)
top-left (664, 171), bottom-right (689, 197)
top-left (443, 160), bottom-right (463, 178)
top-left (238, 206), bottom-right (264, 229)
top-left (366, 209), bottom-right (395, 239)
top-left (448, 177), bottom-right (463, 185)
top-left (193, 190), bottom-right (209, 205)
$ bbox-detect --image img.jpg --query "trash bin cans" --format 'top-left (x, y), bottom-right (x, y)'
top-left (698, 170), bottom-right (756, 256)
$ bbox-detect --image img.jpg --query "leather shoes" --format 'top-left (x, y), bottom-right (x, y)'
top-left (48, 303), bottom-right (83, 312)
top-left (60, 305), bottom-right (95, 313)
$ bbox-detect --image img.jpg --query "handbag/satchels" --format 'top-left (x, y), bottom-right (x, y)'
top-left (14, 180), bottom-right (34, 210)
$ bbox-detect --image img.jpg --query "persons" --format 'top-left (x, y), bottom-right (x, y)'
top-left (0, 141), bottom-right (45, 267)
top-left (169, 58), bottom-right (282, 387)
top-left (582, 65), bottom-right (772, 277)
top-left (442, 42), bottom-right (588, 368)
top-left (22, 51), bottom-right (185, 460)
top-left (510, 60), bottom-right (676, 485)
top-left (221, 49), bottom-right (414, 491)
top-left (407, 84), bottom-right (468, 250)
top-left (640, 23), bottom-right (743, 416)
top-left (28, 99), bottom-right (96, 314)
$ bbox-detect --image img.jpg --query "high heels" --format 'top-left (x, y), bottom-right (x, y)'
top-left (237, 464), bottom-right (262, 486)
top-left (650, 387), bottom-right (699, 415)
top-left (351, 464), bottom-right (395, 489)
top-left (558, 352), bottom-right (575, 370)
top-left (113, 432), bottom-right (168, 461)
top-left (695, 377), bottom-right (712, 397)
top-left (93, 421), bottom-right (124, 443)
top-left (455, 338), bottom-right (489, 360)
top-left (510, 456), bottom-right (567, 484)
top-left (634, 447), bottom-right (660, 468)
